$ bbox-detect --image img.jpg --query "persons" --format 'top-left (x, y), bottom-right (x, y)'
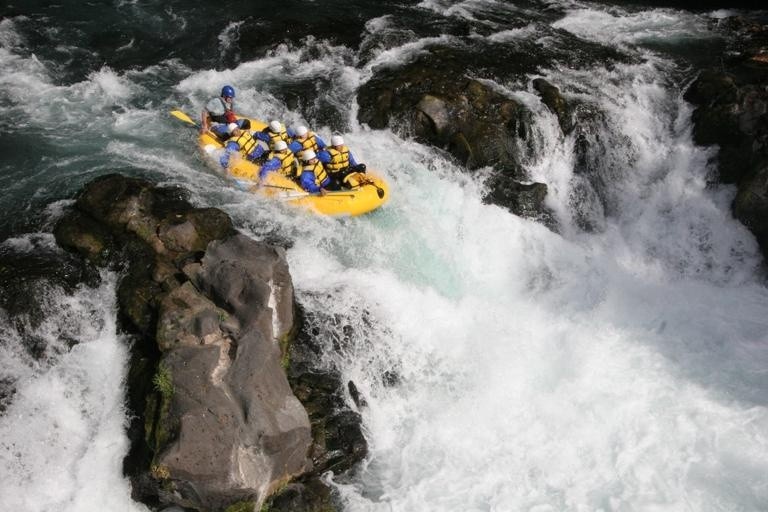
top-left (200, 85), bottom-right (251, 140)
top-left (288, 126), bottom-right (326, 166)
top-left (301, 149), bottom-right (360, 197)
top-left (316, 136), bottom-right (366, 183)
top-left (220, 123), bottom-right (269, 176)
top-left (253, 120), bottom-right (292, 150)
top-left (259, 141), bottom-right (302, 187)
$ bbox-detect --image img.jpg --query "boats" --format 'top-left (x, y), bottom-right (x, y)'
top-left (198, 115), bottom-right (390, 220)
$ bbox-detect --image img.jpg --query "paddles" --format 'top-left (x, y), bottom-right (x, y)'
top-left (236, 177), bottom-right (309, 189)
top-left (205, 144), bottom-right (241, 186)
top-left (170, 111), bottom-right (224, 146)
top-left (278, 188), bottom-right (361, 202)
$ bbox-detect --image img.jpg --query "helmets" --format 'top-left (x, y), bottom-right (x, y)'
top-left (295, 126), bottom-right (308, 137)
top-left (331, 136), bottom-right (345, 147)
top-left (268, 121), bottom-right (281, 134)
top-left (273, 141), bottom-right (287, 151)
top-left (222, 85), bottom-right (236, 98)
top-left (302, 150), bottom-right (316, 162)
top-left (227, 123), bottom-right (239, 134)
